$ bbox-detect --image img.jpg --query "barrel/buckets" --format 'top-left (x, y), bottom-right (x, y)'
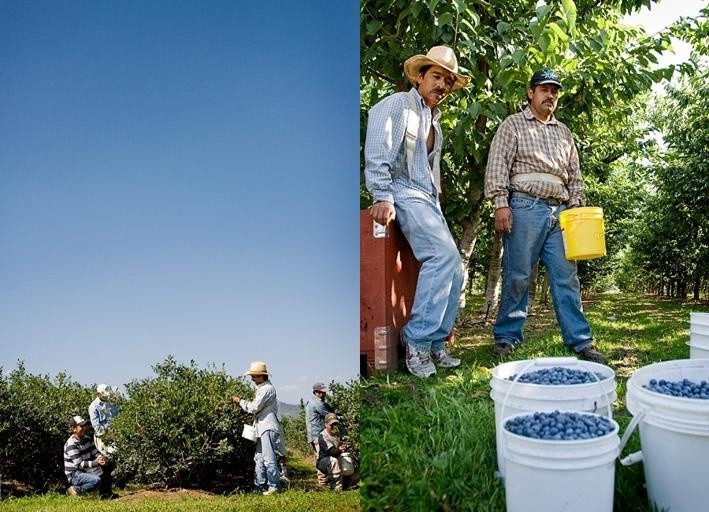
top-left (625, 361), bottom-right (709, 511)
top-left (338, 443), bottom-right (355, 477)
top-left (494, 358), bottom-right (620, 512)
top-left (491, 358), bottom-right (617, 484)
top-left (688, 309), bottom-right (709, 360)
top-left (490, 391), bottom-right (617, 479)
top-left (559, 207), bottom-right (607, 260)
top-left (242, 413), bottom-right (257, 445)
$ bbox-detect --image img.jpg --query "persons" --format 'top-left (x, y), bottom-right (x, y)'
top-left (484, 71), bottom-right (610, 365)
top-left (364, 43), bottom-right (466, 379)
top-left (316, 412), bottom-right (355, 493)
top-left (89, 384), bottom-right (121, 461)
top-left (305, 381), bottom-right (334, 484)
top-left (64, 416), bottom-right (119, 499)
top-left (232, 361), bottom-right (281, 495)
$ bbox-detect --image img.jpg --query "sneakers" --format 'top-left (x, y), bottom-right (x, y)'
top-left (68, 486), bottom-right (79, 496)
top-left (401, 327), bottom-right (437, 377)
top-left (429, 348), bottom-right (461, 367)
top-left (100, 492), bottom-right (119, 499)
top-left (262, 486), bottom-right (280, 495)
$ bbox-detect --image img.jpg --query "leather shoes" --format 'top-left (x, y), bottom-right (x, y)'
top-left (493, 343), bottom-right (511, 358)
top-left (580, 346), bottom-right (604, 360)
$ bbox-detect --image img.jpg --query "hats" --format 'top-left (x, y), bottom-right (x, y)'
top-left (71, 416), bottom-right (87, 425)
top-left (312, 382), bottom-right (329, 393)
top-left (531, 70), bottom-right (563, 89)
top-left (404, 45), bottom-right (472, 92)
top-left (246, 362), bottom-right (269, 375)
top-left (97, 385), bottom-right (113, 398)
top-left (324, 413), bottom-right (339, 425)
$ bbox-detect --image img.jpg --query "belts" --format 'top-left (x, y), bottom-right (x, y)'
top-left (512, 192), bottom-right (567, 206)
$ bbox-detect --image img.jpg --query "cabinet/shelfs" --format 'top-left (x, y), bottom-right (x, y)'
top-left (360, 209), bottom-right (456, 379)
